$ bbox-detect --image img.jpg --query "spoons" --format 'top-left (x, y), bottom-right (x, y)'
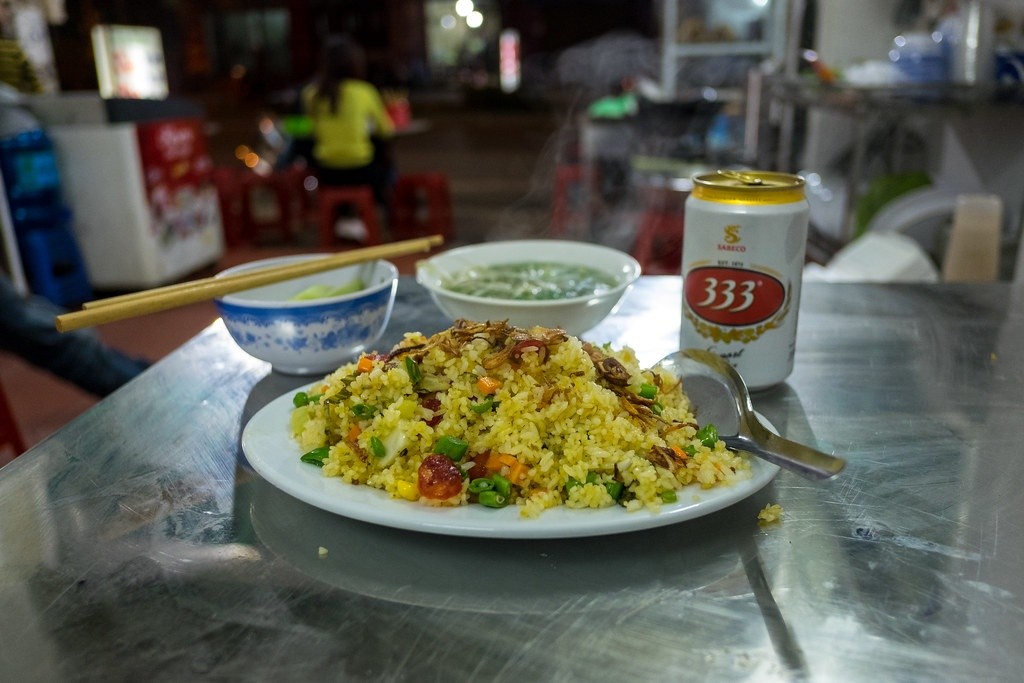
top-left (652, 348), bottom-right (848, 484)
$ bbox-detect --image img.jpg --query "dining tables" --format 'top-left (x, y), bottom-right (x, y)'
top-left (1, 269), bottom-right (1024, 682)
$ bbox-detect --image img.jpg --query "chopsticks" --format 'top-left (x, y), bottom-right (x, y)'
top-left (54, 233), bottom-right (442, 334)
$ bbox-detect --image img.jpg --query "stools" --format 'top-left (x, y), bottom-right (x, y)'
top-left (391, 174), bottom-right (451, 256)
top-left (632, 209), bottom-right (686, 275)
top-left (313, 186), bottom-right (376, 256)
top-left (216, 166), bottom-right (285, 252)
top-left (551, 165), bottom-right (600, 240)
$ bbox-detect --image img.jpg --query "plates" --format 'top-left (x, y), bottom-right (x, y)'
top-left (240, 366), bottom-right (787, 539)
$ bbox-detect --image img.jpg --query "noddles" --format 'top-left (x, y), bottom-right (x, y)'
top-left (415, 258), bottom-right (593, 301)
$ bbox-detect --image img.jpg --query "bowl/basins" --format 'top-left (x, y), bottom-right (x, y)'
top-left (416, 238), bottom-right (644, 337)
top-left (213, 254), bottom-right (401, 379)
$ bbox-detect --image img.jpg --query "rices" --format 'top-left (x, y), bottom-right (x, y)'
top-left (292, 317), bottom-right (785, 523)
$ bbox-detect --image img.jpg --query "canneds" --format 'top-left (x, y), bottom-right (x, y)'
top-left (679, 170), bottom-right (809, 392)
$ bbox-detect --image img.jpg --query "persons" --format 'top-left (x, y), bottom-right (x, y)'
top-left (302, 36), bottom-right (398, 242)
